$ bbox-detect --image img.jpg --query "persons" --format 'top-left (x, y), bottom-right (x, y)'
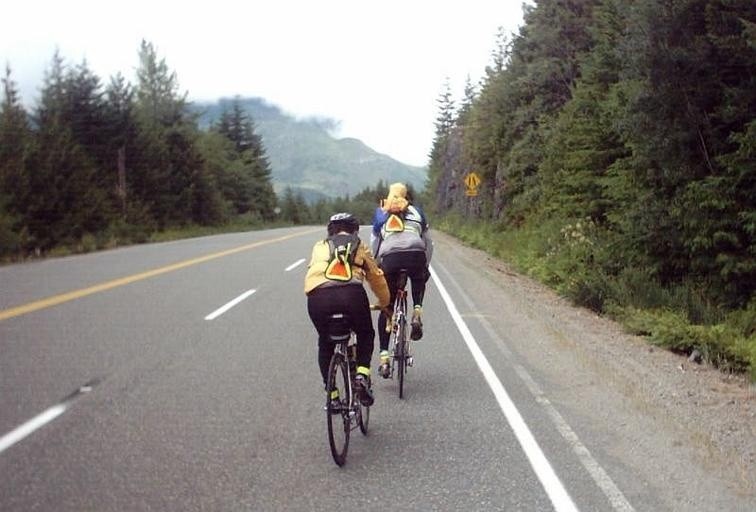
top-left (304, 210), bottom-right (392, 412)
top-left (368, 183), bottom-right (433, 379)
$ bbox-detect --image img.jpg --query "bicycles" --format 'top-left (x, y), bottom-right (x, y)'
top-left (323, 303), bottom-right (387, 465)
top-left (376, 266), bottom-right (418, 397)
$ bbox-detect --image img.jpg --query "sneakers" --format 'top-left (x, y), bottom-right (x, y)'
top-left (379, 364), bottom-right (391, 378)
top-left (410, 317), bottom-right (422, 341)
top-left (355, 375), bottom-right (374, 407)
top-left (325, 401), bottom-right (342, 413)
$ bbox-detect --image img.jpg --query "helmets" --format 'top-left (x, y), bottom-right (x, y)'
top-left (328, 212), bottom-right (359, 233)
